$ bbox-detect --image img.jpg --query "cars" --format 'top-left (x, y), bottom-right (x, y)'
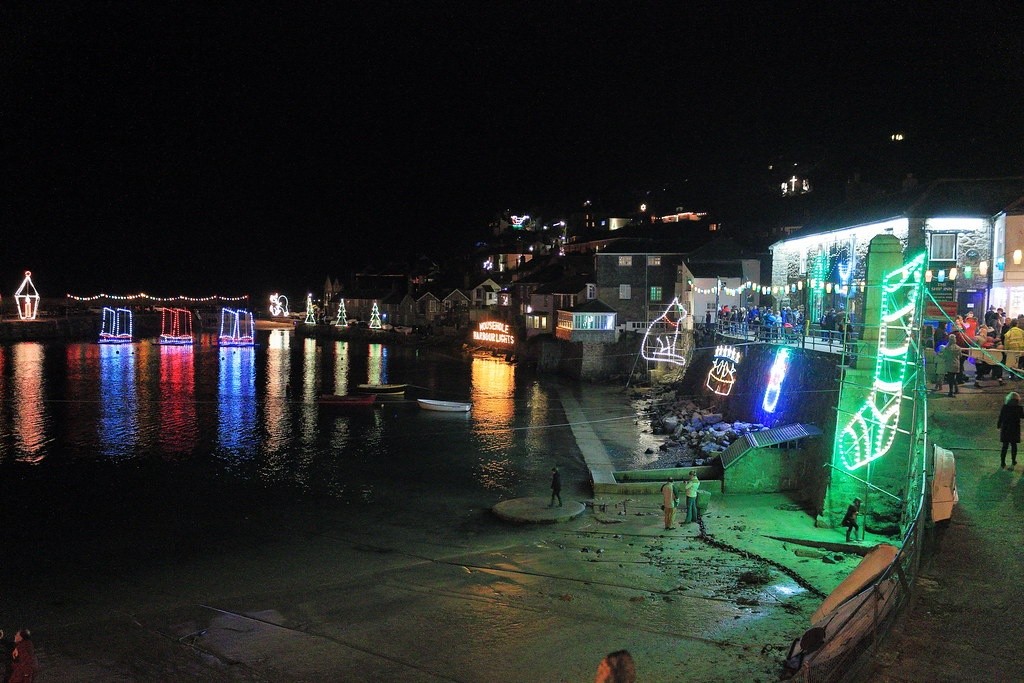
top-left (145, 305), bottom-right (165, 313)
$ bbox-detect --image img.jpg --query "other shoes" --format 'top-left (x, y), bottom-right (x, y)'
top-left (665, 526), bottom-right (676, 530)
top-left (846, 538), bottom-right (852, 542)
top-left (1012, 461), bottom-right (1017, 465)
top-left (681, 521), bottom-right (692, 525)
top-left (939, 386), bottom-right (942, 390)
top-left (691, 519), bottom-right (696, 522)
top-left (999, 379), bottom-right (1007, 385)
top-left (958, 381), bottom-right (965, 384)
top-left (973, 382), bottom-right (981, 388)
top-left (857, 538), bottom-right (863, 541)
top-left (948, 393), bottom-right (955, 397)
top-left (1001, 462), bottom-right (1006, 467)
top-left (976, 376), bottom-right (985, 381)
top-left (548, 504), bottom-right (554, 507)
top-left (557, 504), bottom-right (562, 507)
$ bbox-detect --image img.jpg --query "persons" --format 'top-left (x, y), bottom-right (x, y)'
top-left (935, 305), bottom-right (1024, 396)
top-left (820, 308), bottom-right (853, 345)
top-left (997, 392), bottom-right (1024, 467)
top-left (748, 305), bottom-right (805, 342)
top-left (661, 477), bottom-right (680, 529)
top-left (7, 629), bottom-right (35, 683)
top-left (548, 468), bottom-right (562, 508)
top-left (684, 471), bottom-right (700, 523)
top-left (846, 498), bottom-right (862, 542)
top-left (706, 311), bottom-right (712, 326)
top-left (719, 305), bottom-right (746, 335)
top-left (596, 650), bottom-right (635, 683)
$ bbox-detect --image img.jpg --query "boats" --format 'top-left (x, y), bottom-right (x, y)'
top-left (356, 383), bottom-right (407, 395)
top-left (347, 319), bottom-right (366, 327)
top-left (315, 393), bottom-right (378, 405)
top-left (931, 443), bottom-right (959, 522)
top-left (780, 541), bottom-right (905, 671)
top-left (416, 398), bottom-right (472, 412)
top-left (381, 322), bottom-right (393, 331)
top-left (394, 325), bottom-right (413, 334)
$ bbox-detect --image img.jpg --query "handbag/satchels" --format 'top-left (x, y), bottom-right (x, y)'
top-left (963, 358), bottom-right (977, 371)
